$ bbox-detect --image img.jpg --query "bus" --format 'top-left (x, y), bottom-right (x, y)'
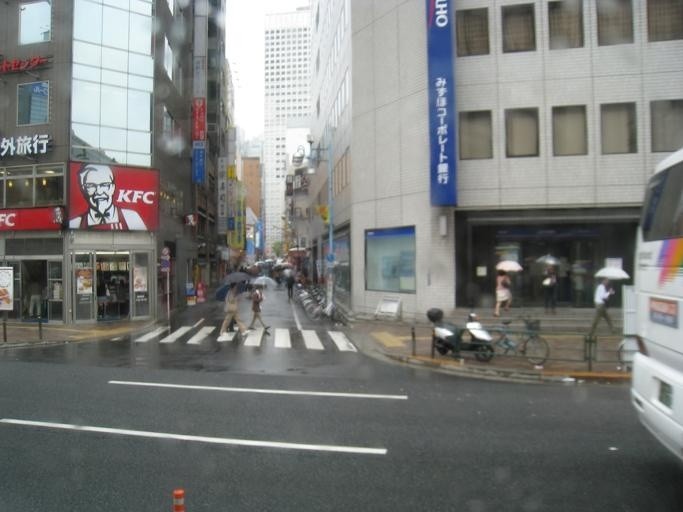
top-left (628, 146), bottom-right (682, 463)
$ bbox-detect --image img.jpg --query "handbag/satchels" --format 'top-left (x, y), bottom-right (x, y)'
top-left (501, 277), bottom-right (511, 289)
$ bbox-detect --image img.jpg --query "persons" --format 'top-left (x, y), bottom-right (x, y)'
top-left (26, 276), bottom-right (40, 316)
top-left (494, 269), bottom-right (513, 317)
top-left (517, 254), bottom-right (588, 317)
top-left (213, 256), bottom-right (305, 336)
top-left (66, 164), bottom-right (148, 231)
top-left (589, 280), bottom-right (615, 338)
top-left (98, 278), bottom-right (127, 317)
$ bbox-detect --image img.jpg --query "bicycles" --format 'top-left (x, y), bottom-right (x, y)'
top-left (485, 314), bottom-right (550, 364)
top-left (293, 284), bottom-right (343, 322)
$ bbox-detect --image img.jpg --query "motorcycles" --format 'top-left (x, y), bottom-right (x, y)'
top-left (426, 306), bottom-right (494, 362)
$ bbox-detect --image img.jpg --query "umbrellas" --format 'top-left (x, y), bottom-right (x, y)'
top-left (593, 265), bottom-right (632, 289)
top-left (496, 260), bottom-right (524, 273)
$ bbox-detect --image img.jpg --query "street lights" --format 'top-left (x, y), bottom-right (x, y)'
top-left (292, 132), bottom-right (335, 322)
top-left (281, 214), bottom-right (300, 273)
